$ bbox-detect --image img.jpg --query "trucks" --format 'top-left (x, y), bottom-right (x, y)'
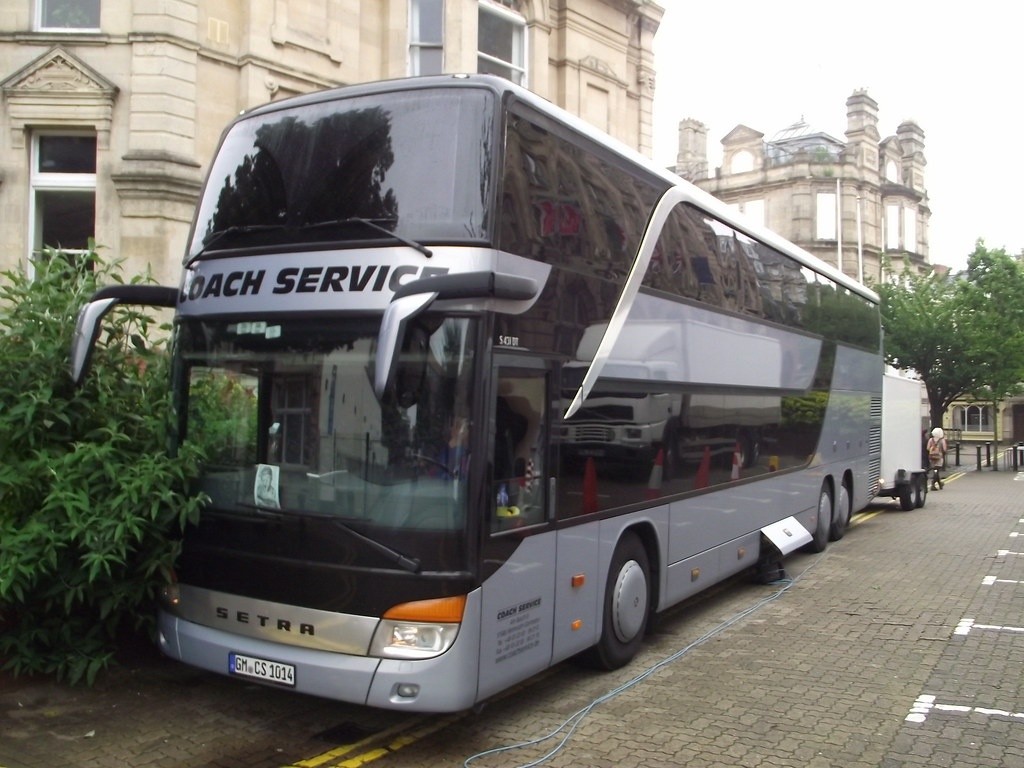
top-left (546, 316), bottom-right (786, 476)
top-left (870, 379), bottom-right (933, 512)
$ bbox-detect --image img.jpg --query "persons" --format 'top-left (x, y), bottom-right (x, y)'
top-left (425, 412), bottom-right (510, 505)
top-left (921, 429), bottom-right (931, 493)
top-left (926, 427), bottom-right (946, 491)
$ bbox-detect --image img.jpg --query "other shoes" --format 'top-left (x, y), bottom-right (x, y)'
top-left (931, 486), bottom-right (938, 491)
top-left (939, 482), bottom-right (945, 489)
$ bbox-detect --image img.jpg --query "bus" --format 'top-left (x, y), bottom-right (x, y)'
top-left (68, 66), bottom-right (888, 712)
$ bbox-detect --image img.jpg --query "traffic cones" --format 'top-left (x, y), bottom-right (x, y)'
top-left (694, 444), bottom-right (712, 490)
top-left (730, 440), bottom-right (742, 484)
top-left (645, 446), bottom-right (665, 502)
top-left (581, 456), bottom-right (600, 517)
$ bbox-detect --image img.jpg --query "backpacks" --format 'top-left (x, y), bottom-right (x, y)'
top-left (928, 436), bottom-right (944, 461)
